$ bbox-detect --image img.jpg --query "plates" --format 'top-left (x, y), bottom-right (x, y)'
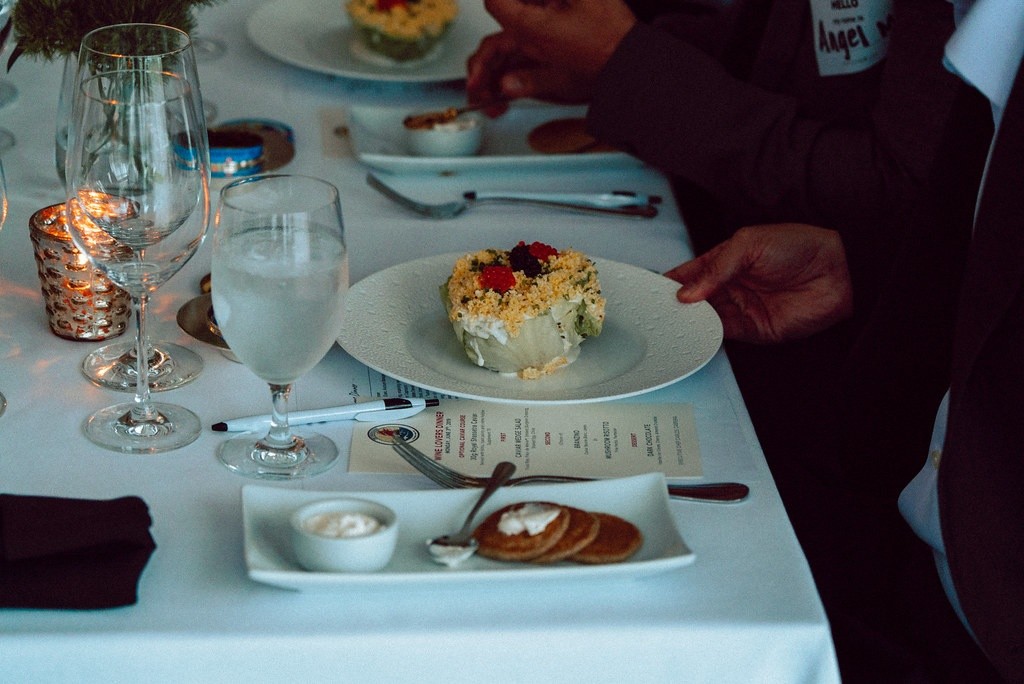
top-left (346, 101), bottom-right (642, 174)
top-left (247, 1), bottom-right (505, 82)
top-left (241, 470), bottom-right (697, 592)
top-left (334, 250), bottom-right (725, 403)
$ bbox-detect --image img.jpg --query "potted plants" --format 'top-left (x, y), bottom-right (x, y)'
top-left (11, 1), bottom-right (203, 196)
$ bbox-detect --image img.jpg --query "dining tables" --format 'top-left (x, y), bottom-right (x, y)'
top-left (0, 0), bottom-right (847, 680)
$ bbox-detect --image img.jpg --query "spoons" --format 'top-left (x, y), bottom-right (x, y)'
top-left (423, 461), bottom-right (517, 566)
top-left (401, 102), bottom-right (492, 129)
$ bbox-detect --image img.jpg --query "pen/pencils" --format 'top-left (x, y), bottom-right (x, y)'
top-left (462, 189), bottom-right (663, 208)
top-left (211, 398), bottom-right (439, 433)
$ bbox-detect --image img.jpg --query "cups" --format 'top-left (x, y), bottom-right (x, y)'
top-left (29, 197), bottom-right (139, 341)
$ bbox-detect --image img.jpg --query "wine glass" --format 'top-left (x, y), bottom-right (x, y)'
top-left (65, 71), bottom-right (210, 457)
top-left (68, 23), bottom-right (206, 393)
top-left (210, 175), bottom-right (348, 481)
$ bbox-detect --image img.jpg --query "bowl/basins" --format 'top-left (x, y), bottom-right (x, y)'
top-left (406, 108), bottom-right (492, 154)
top-left (176, 129), bottom-right (263, 177)
top-left (287, 496), bottom-right (401, 574)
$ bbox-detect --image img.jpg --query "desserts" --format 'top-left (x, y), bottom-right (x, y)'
top-left (437, 240), bottom-right (607, 379)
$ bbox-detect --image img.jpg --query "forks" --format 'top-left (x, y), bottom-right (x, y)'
top-left (390, 436), bottom-right (750, 502)
top-left (365, 168), bottom-right (660, 220)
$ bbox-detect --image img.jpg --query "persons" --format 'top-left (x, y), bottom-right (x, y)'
top-left (461, 0), bottom-right (1024, 176)
top-left (668, 0), bottom-right (1024, 683)
